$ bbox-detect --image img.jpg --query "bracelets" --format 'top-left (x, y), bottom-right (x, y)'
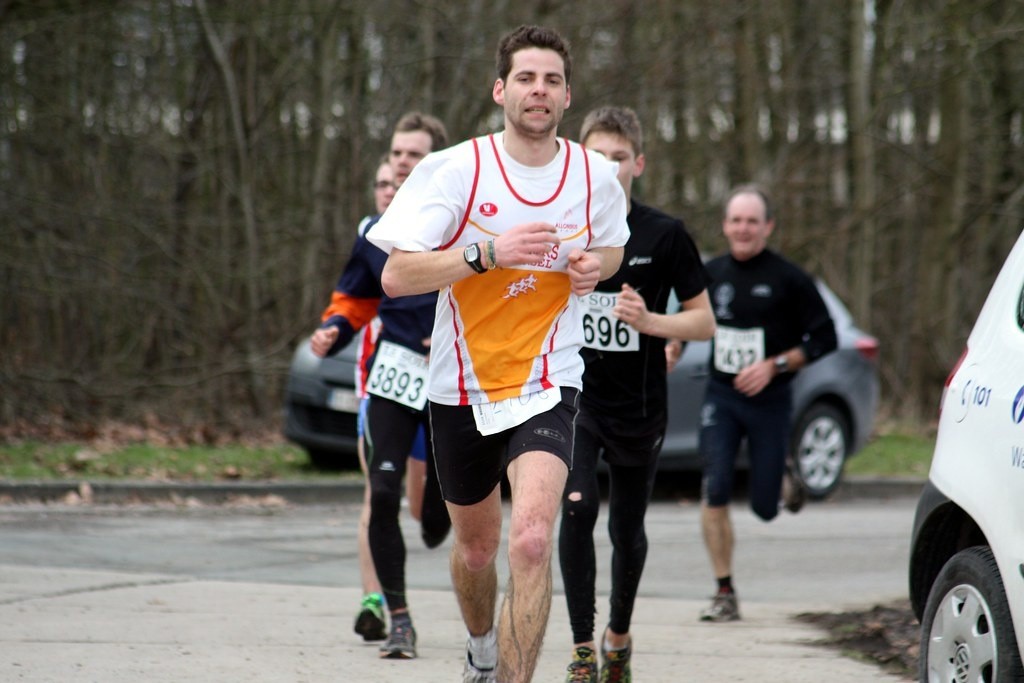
top-left (484, 239), bottom-right (497, 270)
top-left (774, 355), bottom-right (788, 372)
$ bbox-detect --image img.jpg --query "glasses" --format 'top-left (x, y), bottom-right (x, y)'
top-left (373, 179), bottom-right (400, 189)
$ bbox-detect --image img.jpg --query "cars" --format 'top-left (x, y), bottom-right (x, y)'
top-left (281, 254), bottom-right (880, 501)
top-left (909, 230), bottom-right (1024, 683)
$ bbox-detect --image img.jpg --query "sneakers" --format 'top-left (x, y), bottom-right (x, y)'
top-left (564, 646), bottom-right (598, 683)
top-left (462, 640), bottom-right (499, 683)
top-left (354, 606), bottom-right (388, 641)
top-left (378, 624), bottom-right (417, 658)
top-left (699, 592), bottom-right (741, 622)
top-left (598, 626), bottom-right (633, 683)
top-left (783, 453), bottom-right (807, 514)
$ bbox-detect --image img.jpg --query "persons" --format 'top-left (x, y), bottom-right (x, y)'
top-left (354, 155), bottom-right (427, 641)
top-left (558, 104), bottom-right (716, 683)
top-left (364, 23), bottom-right (632, 683)
top-left (310, 113), bottom-right (452, 661)
top-left (665, 187), bottom-right (838, 623)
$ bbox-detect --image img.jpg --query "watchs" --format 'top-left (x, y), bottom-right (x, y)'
top-left (464, 243), bottom-right (487, 273)
